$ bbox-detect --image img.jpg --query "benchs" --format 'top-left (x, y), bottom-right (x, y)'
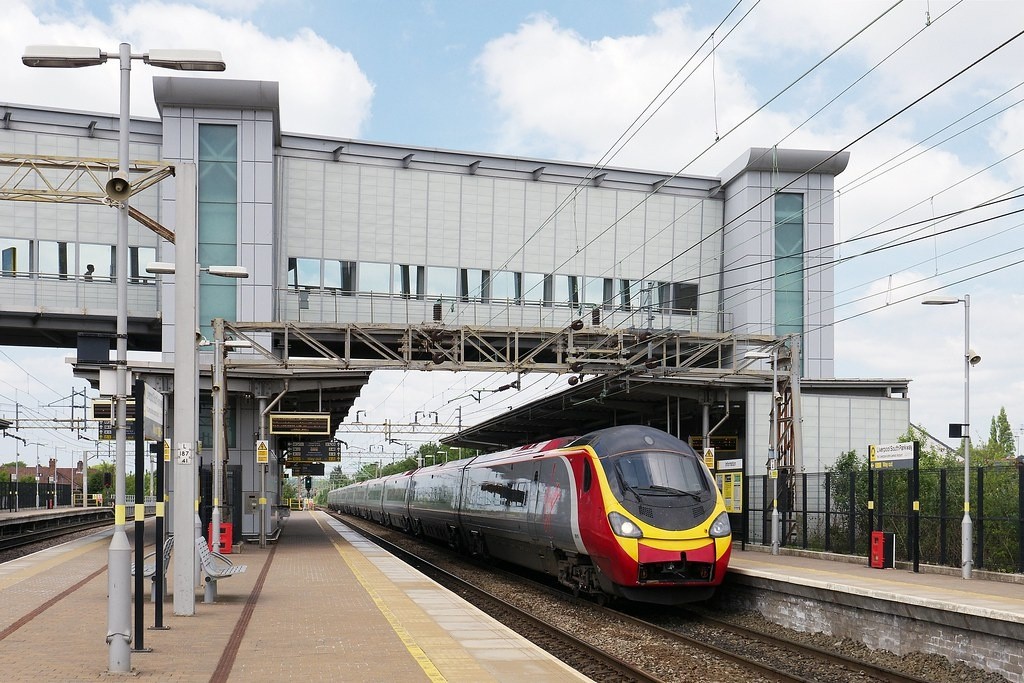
top-left (130, 537), bottom-right (175, 601)
top-left (195, 536), bottom-right (247, 604)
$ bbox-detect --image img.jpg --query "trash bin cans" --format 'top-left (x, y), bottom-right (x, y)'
top-left (868, 531), bottom-right (894, 569)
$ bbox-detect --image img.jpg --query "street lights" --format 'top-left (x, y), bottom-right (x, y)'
top-left (34, 438), bottom-right (47, 510)
top-left (53, 444), bottom-right (66, 509)
top-left (20, 40), bottom-right (228, 673)
top-left (70, 449), bottom-right (81, 507)
top-left (922, 292), bottom-right (975, 582)
top-left (15, 438), bottom-right (30, 513)
top-left (744, 349), bottom-right (781, 555)
top-left (142, 259), bottom-right (251, 605)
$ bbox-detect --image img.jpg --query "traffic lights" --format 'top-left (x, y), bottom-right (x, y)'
top-left (304, 477), bottom-right (311, 491)
top-left (104, 472), bottom-right (112, 488)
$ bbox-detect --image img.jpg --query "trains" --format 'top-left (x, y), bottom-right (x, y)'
top-left (327, 425), bottom-right (732, 606)
